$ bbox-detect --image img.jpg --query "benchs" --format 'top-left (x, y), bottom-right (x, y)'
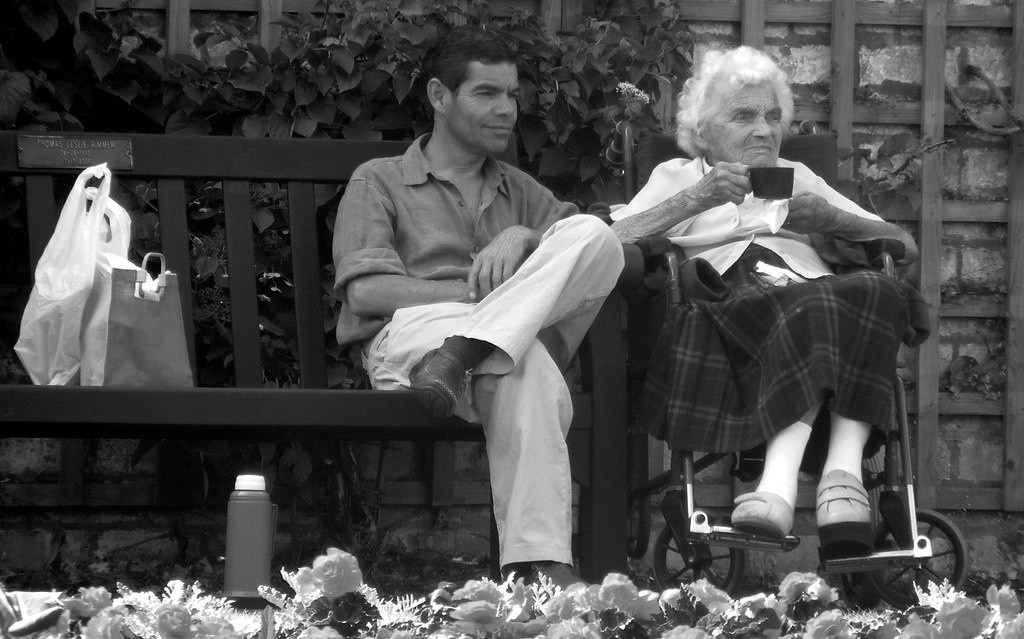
top-left (0, 130), bottom-right (645, 585)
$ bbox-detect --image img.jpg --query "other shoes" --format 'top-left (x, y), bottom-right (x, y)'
top-left (523, 564), bottom-right (597, 591)
top-left (408, 345), bottom-right (473, 421)
top-left (730, 492), bottom-right (795, 540)
top-left (816, 470), bottom-right (874, 556)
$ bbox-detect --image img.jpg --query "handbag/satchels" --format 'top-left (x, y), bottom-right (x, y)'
top-left (14, 162), bottom-right (132, 385)
top-left (80, 252), bottom-right (195, 389)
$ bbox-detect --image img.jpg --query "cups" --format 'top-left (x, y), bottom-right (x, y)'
top-left (746, 166), bottom-right (795, 199)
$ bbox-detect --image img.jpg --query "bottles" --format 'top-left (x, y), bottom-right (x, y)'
top-left (136, 270), bottom-right (166, 299)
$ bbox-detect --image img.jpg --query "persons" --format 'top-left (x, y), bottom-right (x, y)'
top-left (588, 43), bottom-right (933, 542)
top-left (330, 24), bottom-right (626, 602)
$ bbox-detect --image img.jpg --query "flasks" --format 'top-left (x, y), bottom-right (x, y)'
top-left (222, 475), bottom-right (279, 598)
